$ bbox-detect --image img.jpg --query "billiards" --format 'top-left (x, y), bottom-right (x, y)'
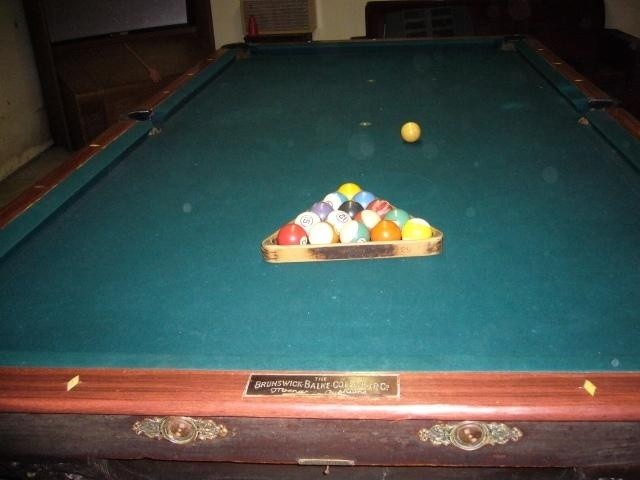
top-left (277, 183), bottom-right (433, 245)
top-left (401, 122), bottom-right (421, 142)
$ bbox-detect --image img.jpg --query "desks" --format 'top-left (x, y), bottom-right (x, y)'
top-left (0, 30), bottom-right (640, 479)
